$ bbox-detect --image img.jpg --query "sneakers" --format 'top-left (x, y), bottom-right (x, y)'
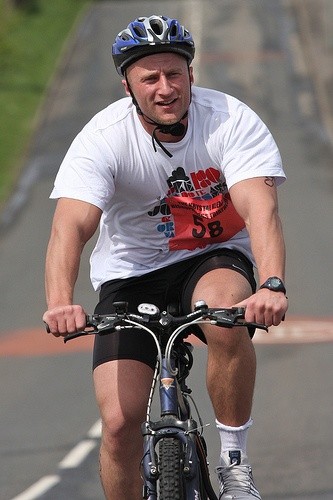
top-left (214, 449), bottom-right (262, 500)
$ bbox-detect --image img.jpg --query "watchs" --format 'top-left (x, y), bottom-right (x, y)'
top-left (259, 276), bottom-right (287, 295)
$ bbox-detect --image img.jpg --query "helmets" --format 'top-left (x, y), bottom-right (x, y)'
top-left (112, 14), bottom-right (195, 77)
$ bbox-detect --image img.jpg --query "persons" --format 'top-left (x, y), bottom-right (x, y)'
top-left (43, 15), bottom-right (289, 500)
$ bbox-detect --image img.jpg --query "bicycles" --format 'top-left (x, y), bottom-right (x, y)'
top-left (43, 303), bottom-right (285, 499)
